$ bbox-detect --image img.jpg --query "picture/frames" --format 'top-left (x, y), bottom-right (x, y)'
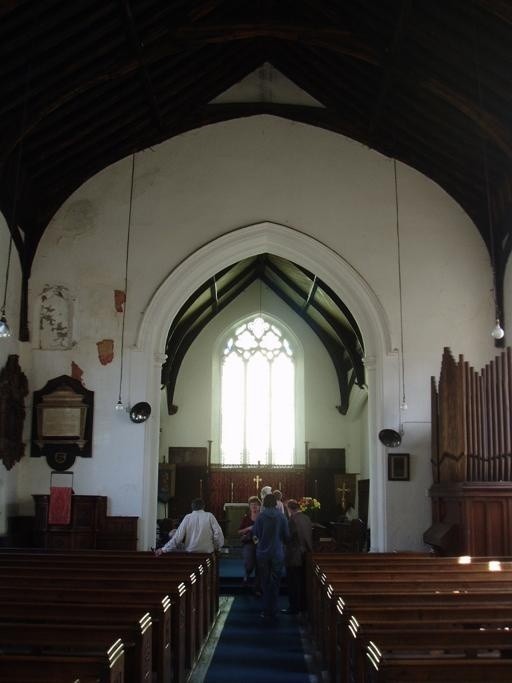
top-left (387, 452), bottom-right (410, 480)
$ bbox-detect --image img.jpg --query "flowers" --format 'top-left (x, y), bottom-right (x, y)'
top-left (298, 495), bottom-right (321, 515)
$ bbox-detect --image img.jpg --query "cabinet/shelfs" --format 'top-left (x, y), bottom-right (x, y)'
top-left (1, 494), bottom-right (138, 547)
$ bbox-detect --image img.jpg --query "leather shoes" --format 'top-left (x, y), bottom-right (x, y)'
top-left (280, 608), bottom-right (296, 613)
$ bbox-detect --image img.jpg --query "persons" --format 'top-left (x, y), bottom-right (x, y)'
top-left (239, 485), bottom-right (313, 624)
top-left (153, 497), bottom-right (225, 558)
